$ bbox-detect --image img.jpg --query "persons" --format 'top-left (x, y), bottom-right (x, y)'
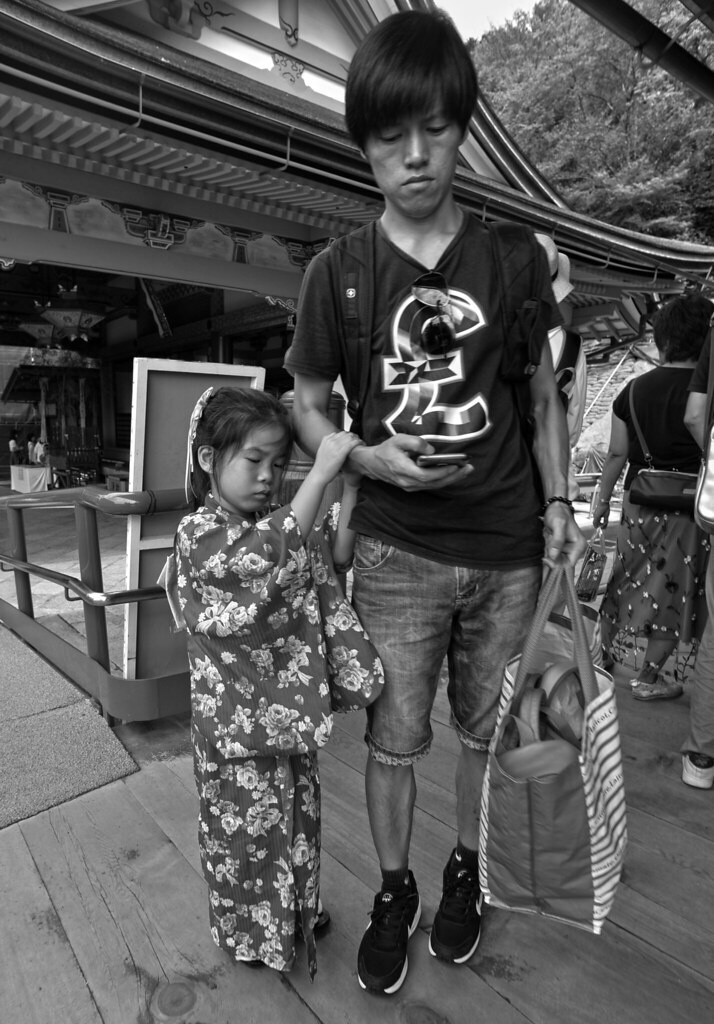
top-left (167, 384), bottom-right (365, 982)
top-left (681, 324), bottom-right (714, 789)
top-left (533, 232), bottom-right (589, 446)
top-left (594, 297), bottom-right (714, 700)
top-left (291, 12), bottom-right (587, 996)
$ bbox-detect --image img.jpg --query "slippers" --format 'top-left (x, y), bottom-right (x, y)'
top-left (240, 907), bottom-right (332, 969)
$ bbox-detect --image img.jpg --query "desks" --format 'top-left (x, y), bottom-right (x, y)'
top-left (10, 465), bottom-right (51, 493)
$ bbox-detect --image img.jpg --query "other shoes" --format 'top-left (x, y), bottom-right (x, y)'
top-left (682, 750), bottom-right (714, 788)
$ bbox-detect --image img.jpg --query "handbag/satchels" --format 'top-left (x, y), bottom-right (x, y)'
top-left (628, 466), bottom-right (699, 507)
top-left (478, 554), bottom-right (628, 935)
top-left (574, 526), bottom-right (609, 602)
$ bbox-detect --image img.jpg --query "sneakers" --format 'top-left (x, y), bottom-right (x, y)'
top-left (603, 651), bottom-right (613, 669)
top-left (428, 847), bottom-right (483, 964)
top-left (357, 870), bottom-right (421, 994)
top-left (630, 674), bottom-right (683, 700)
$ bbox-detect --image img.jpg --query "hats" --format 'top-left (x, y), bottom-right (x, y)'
top-left (535, 232), bottom-right (574, 303)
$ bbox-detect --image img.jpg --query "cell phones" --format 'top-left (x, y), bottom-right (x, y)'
top-left (416, 453), bottom-right (471, 469)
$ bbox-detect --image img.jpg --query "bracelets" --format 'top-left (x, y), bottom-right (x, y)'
top-left (597, 499), bottom-right (610, 503)
top-left (541, 497), bottom-right (575, 516)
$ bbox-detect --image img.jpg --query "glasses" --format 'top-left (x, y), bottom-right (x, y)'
top-left (411, 271), bottom-right (458, 359)
